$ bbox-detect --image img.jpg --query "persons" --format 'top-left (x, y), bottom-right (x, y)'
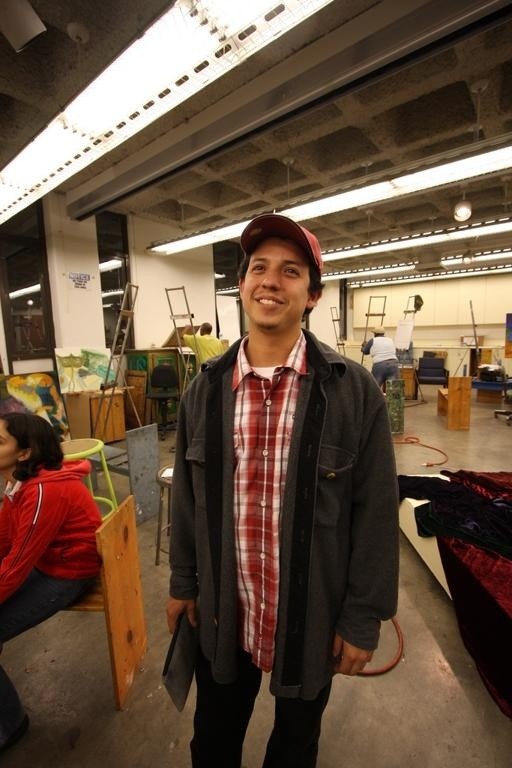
top-left (180, 323), bottom-right (226, 375)
top-left (360, 325), bottom-right (399, 388)
top-left (165, 212), bottom-right (398, 768)
top-left (0, 412), bottom-right (104, 754)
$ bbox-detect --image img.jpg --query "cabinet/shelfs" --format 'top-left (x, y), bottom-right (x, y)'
top-left (124, 347), bottom-right (197, 431)
top-left (399, 367), bottom-right (418, 401)
top-left (62, 390), bottom-right (126, 445)
top-left (470, 348), bottom-right (493, 379)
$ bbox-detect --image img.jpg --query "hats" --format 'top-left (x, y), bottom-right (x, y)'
top-left (370, 325), bottom-right (387, 335)
top-left (237, 210), bottom-right (325, 275)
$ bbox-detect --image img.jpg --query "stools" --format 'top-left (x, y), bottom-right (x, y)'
top-left (154, 463), bottom-right (177, 566)
top-left (58, 437), bottom-right (117, 520)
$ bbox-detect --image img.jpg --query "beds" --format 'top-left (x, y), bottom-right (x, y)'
top-left (398, 468), bottom-right (512, 724)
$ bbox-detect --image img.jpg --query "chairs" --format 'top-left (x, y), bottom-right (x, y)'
top-left (494, 374), bottom-right (512, 426)
top-left (145, 364), bottom-right (181, 441)
top-left (414, 357), bottom-right (450, 400)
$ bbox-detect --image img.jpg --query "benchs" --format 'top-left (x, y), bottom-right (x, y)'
top-left (437, 376), bottom-right (473, 431)
top-left (60, 494), bottom-right (146, 711)
